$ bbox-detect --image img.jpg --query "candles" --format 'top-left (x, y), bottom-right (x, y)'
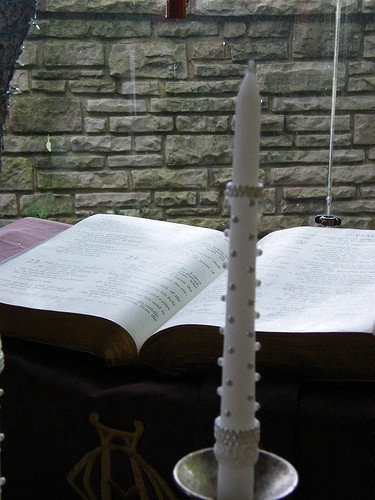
top-left (206, 58), bottom-right (263, 499)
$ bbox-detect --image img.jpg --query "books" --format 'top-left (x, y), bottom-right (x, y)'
top-left (0, 212), bottom-right (374, 356)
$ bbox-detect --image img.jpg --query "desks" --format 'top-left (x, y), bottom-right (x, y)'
top-left (1, 352), bottom-right (372, 500)
